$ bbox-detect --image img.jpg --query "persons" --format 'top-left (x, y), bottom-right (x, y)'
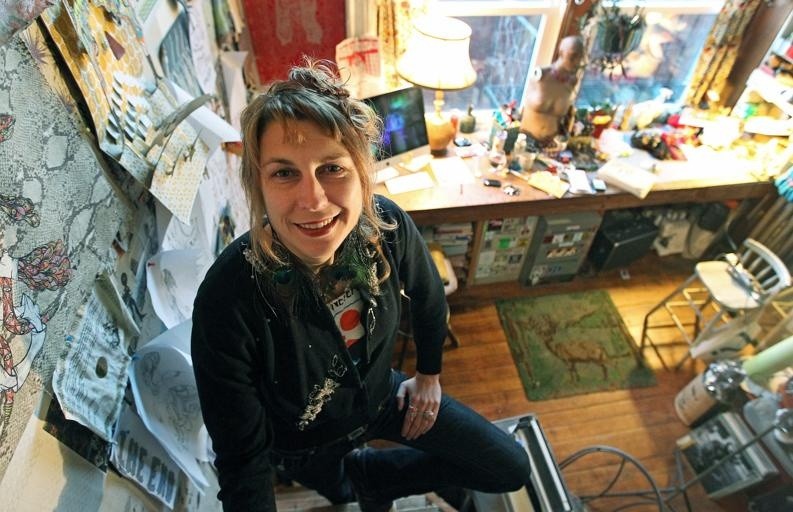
top-left (189, 50), bottom-right (533, 512)
top-left (519, 32), bottom-right (583, 141)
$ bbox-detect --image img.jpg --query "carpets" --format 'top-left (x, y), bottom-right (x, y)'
top-left (490, 287), bottom-right (656, 400)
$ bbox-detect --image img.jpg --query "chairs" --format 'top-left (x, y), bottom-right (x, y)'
top-left (391, 249), bottom-right (461, 364)
top-left (646, 237), bottom-right (792, 374)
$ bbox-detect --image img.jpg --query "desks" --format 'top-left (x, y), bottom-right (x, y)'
top-left (372, 119), bottom-right (775, 295)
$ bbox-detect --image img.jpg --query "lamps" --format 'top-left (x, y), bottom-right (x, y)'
top-left (395, 16), bottom-right (478, 154)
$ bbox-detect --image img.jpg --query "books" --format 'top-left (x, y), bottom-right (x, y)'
top-left (526, 168), bottom-right (568, 202)
top-left (672, 411), bottom-right (780, 501)
top-left (434, 222), bottom-right (473, 286)
top-left (597, 157), bottom-right (659, 199)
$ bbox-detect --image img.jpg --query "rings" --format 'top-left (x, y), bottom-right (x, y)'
top-left (405, 405), bottom-right (417, 418)
top-left (422, 410), bottom-right (433, 419)
